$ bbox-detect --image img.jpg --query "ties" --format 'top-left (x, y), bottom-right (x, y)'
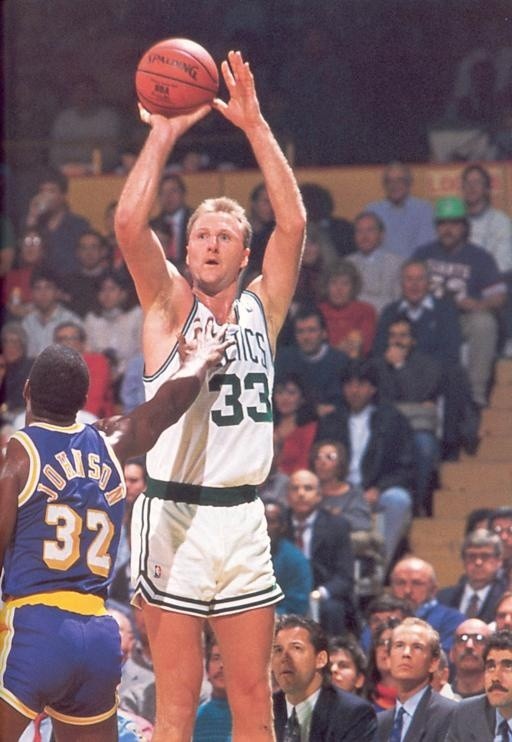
top-left (500, 721), bottom-right (509, 742)
top-left (282, 706), bottom-right (302, 742)
top-left (387, 706), bottom-right (406, 742)
top-left (465, 594), bottom-right (481, 619)
top-left (295, 521), bottom-right (307, 552)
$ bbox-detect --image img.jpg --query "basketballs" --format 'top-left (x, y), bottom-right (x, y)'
top-left (136, 38), bottom-right (220, 118)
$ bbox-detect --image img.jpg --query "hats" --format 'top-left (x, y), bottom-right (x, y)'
top-left (431, 197), bottom-right (468, 222)
